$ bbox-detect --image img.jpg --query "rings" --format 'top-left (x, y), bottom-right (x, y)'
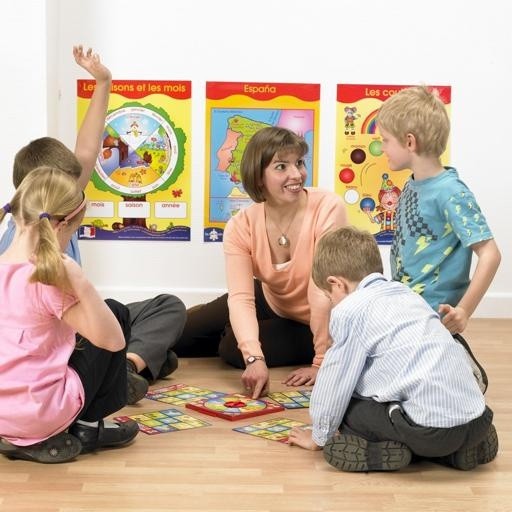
top-left (303, 375), bottom-right (309, 380)
top-left (245, 385), bottom-right (252, 391)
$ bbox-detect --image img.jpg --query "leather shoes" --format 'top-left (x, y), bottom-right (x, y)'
top-left (1, 431), bottom-right (82, 464)
top-left (68, 419), bottom-right (139, 454)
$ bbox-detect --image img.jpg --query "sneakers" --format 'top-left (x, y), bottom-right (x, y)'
top-left (453, 424), bottom-right (498, 470)
top-left (323, 434), bottom-right (411, 472)
top-left (453, 332), bottom-right (488, 395)
top-left (127, 370), bottom-right (149, 406)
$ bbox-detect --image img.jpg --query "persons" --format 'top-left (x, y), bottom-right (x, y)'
top-left (0, 166), bottom-right (140, 464)
top-left (288, 223), bottom-right (500, 474)
top-left (372, 84), bottom-right (505, 390)
top-left (169, 125), bottom-right (349, 399)
top-left (0, 42), bottom-right (189, 404)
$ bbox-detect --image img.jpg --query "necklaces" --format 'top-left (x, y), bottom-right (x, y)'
top-left (261, 200), bottom-right (300, 246)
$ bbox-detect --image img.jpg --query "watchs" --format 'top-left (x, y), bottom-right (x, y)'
top-left (242, 355), bottom-right (267, 366)
